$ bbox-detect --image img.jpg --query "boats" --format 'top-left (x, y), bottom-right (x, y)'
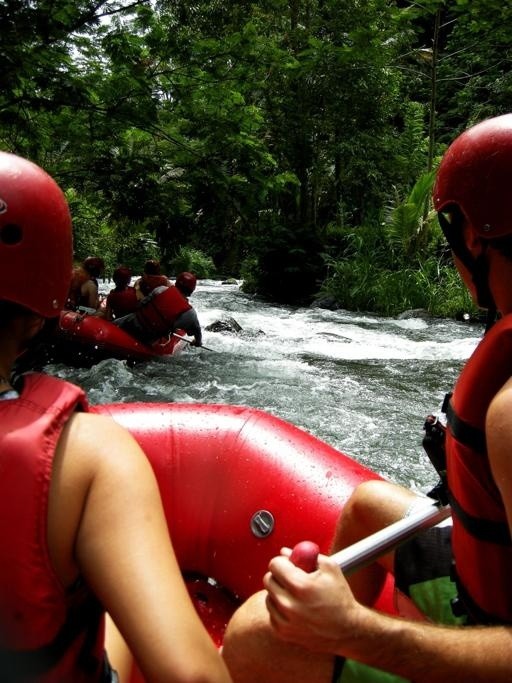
top-left (58, 299), bottom-right (186, 355)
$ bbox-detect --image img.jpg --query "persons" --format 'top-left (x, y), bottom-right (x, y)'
top-left (0, 151), bottom-right (232, 683)
top-left (222, 112), bottom-right (512, 683)
top-left (67, 256), bottom-right (104, 312)
top-left (102, 259), bottom-right (202, 355)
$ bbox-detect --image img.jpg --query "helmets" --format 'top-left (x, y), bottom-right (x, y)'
top-left (175, 272), bottom-right (197, 295)
top-left (111, 267), bottom-right (132, 285)
top-left (431, 111), bottom-right (512, 247)
top-left (0, 148), bottom-right (77, 322)
top-left (83, 256), bottom-right (104, 278)
top-left (143, 259), bottom-right (161, 275)
